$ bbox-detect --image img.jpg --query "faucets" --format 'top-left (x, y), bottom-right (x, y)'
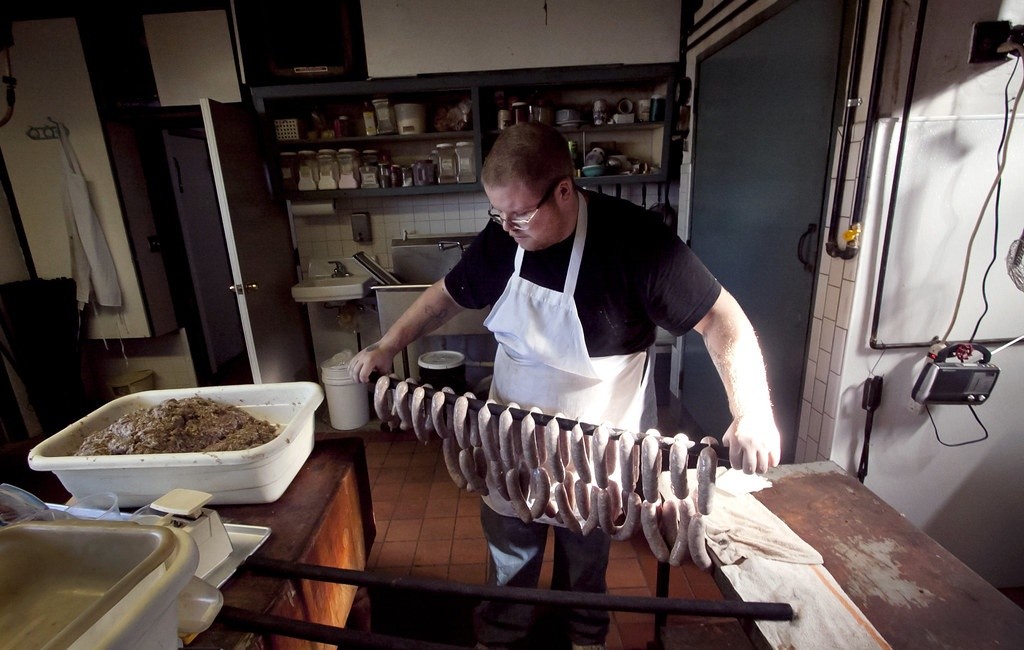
top-left (437, 241), bottom-right (466, 258)
top-left (327, 259), bottom-right (353, 278)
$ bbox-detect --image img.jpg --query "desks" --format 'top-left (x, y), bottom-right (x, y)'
top-left (643, 459), bottom-right (1024, 650)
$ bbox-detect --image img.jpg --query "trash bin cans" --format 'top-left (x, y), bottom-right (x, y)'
top-left (319, 359), bottom-right (371, 432)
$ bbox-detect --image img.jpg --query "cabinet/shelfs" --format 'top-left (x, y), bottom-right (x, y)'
top-left (0, 439), bottom-right (381, 650)
top-left (251, 61), bottom-right (684, 201)
top-left (1, 15), bottom-right (178, 343)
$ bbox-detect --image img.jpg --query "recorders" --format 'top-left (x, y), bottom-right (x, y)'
top-left (910, 362), bottom-right (1000, 406)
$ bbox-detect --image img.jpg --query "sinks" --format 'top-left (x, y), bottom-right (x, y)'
top-left (290, 275), bottom-right (376, 302)
top-left (370, 273), bottom-right (508, 339)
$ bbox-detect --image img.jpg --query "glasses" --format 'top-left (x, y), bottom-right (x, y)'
top-left (489, 183), bottom-right (556, 229)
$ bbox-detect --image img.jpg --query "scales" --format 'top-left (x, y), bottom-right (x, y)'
top-left (150, 487), bottom-right (235, 585)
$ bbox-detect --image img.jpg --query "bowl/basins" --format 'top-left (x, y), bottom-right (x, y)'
top-left (64, 492), bottom-right (122, 521)
top-left (582, 165), bottom-right (605, 177)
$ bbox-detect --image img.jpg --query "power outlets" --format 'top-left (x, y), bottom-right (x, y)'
top-left (967, 21), bottom-right (1012, 63)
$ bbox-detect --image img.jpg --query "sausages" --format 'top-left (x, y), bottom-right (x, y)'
top-left (374, 373), bottom-right (719, 572)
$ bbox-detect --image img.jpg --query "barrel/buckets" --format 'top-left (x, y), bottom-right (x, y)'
top-left (108, 370), bottom-right (154, 400)
top-left (418, 350), bottom-right (467, 396)
top-left (320, 358), bottom-right (370, 431)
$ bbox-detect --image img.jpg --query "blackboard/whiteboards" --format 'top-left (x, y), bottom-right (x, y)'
top-left (868, 113), bottom-right (1024, 350)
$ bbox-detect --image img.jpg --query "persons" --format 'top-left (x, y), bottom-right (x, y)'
top-left (345, 120), bottom-right (788, 650)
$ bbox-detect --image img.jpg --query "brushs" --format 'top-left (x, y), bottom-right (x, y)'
top-left (858, 376), bottom-right (883, 484)
